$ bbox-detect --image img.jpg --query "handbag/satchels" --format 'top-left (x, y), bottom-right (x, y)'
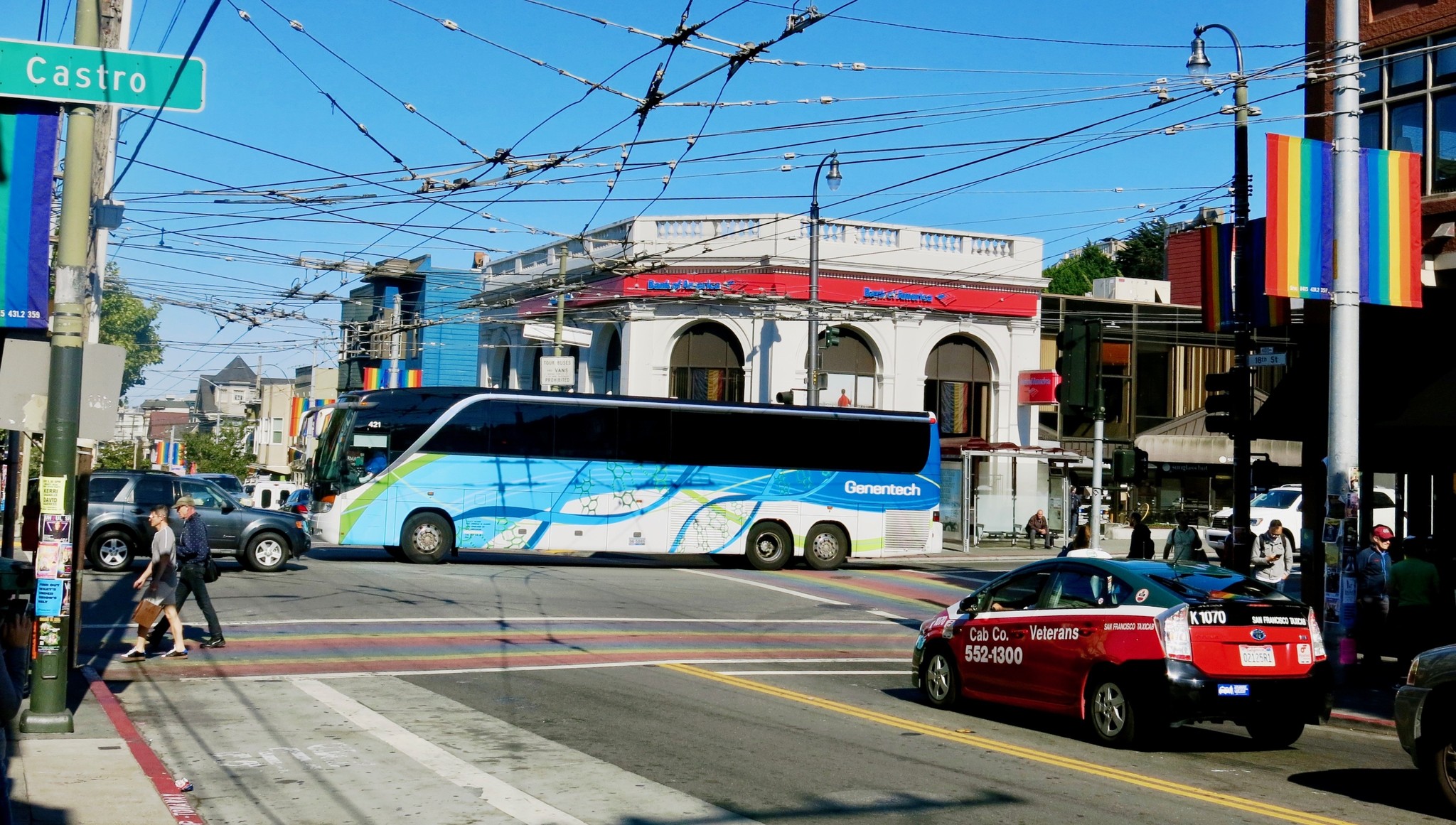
top-left (204, 555), bottom-right (221, 583)
top-left (1194, 547), bottom-right (1210, 564)
top-left (131, 591), bottom-right (164, 629)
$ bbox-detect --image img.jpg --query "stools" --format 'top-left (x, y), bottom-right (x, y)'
top-left (983, 530), bottom-right (1060, 548)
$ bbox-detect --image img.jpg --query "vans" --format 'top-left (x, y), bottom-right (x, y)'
top-left (253, 480), bottom-right (296, 511)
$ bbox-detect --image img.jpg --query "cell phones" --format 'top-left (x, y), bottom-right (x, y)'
top-left (1275, 555), bottom-right (1282, 558)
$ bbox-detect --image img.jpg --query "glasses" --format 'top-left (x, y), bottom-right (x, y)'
top-left (1271, 534), bottom-right (1281, 537)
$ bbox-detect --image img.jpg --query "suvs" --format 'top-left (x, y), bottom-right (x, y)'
top-left (84, 469), bottom-right (311, 573)
top-left (1204, 484), bottom-right (1408, 561)
top-left (180, 473), bottom-right (254, 510)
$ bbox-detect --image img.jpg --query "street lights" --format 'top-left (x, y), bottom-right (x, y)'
top-left (188, 375), bottom-right (222, 446)
top-left (806, 148), bottom-right (843, 407)
top-left (1184, 20), bottom-right (1254, 574)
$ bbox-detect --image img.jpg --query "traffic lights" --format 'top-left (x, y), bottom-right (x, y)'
top-left (1204, 365), bottom-right (1238, 441)
top-left (825, 326), bottom-right (840, 347)
top-left (776, 391), bottom-right (794, 405)
top-left (1134, 447), bottom-right (1148, 488)
top-left (1054, 324), bottom-right (1087, 407)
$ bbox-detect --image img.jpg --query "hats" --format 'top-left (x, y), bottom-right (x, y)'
top-left (1374, 526), bottom-right (1394, 540)
top-left (171, 496), bottom-right (195, 508)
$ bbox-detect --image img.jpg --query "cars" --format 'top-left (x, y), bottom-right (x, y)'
top-left (1394, 643), bottom-right (1456, 820)
top-left (911, 549), bottom-right (1328, 751)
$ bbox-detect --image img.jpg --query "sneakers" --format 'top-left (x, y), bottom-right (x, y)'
top-left (1030, 545), bottom-right (1035, 549)
top-left (121, 646), bottom-right (146, 661)
top-left (161, 648), bottom-right (188, 659)
top-left (1045, 545), bottom-right (1053, 549)
top-left (1392, 683), bottom-right (1401, 690)
top-left (1366, 685), bottom-right (1380, 693)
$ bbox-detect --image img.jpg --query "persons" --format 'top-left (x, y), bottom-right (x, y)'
top-left (1220, 514), bottom-right (1258, 577)
top-left (1126, 512), bottom-right (1151, 559)
top-left (146, 496), bottom-right (226, 649)
top-left (120, 504), bottom-right (188, 662)
top-left (1025, 510), bottom-right (1052, 549)
top-left (1352, 524), bottom-right (1440, 722)
top-left (1250, 519), bottom-right (1294, 594)
top-left (1069, 485), bottom-right (1082, 538)
top-left (991, 574), bottom-right (1047, 611)
top-left (0, 605), bottom-right (33, 825)
top-left (349, 448), bottom-right (387, 485)
top-left (1162, 510), bottom-right (1202, 560)
top-left (1057, 525), bottom-right (1090, 558)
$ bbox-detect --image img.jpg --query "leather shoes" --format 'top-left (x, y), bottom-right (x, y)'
top-left (138, 632), bottom-right (161, 648)
top-left (200, 635), bottom-right (225, 649)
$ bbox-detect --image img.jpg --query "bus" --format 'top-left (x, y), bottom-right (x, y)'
top-left (293, 386), bottom-right (944, 571)
